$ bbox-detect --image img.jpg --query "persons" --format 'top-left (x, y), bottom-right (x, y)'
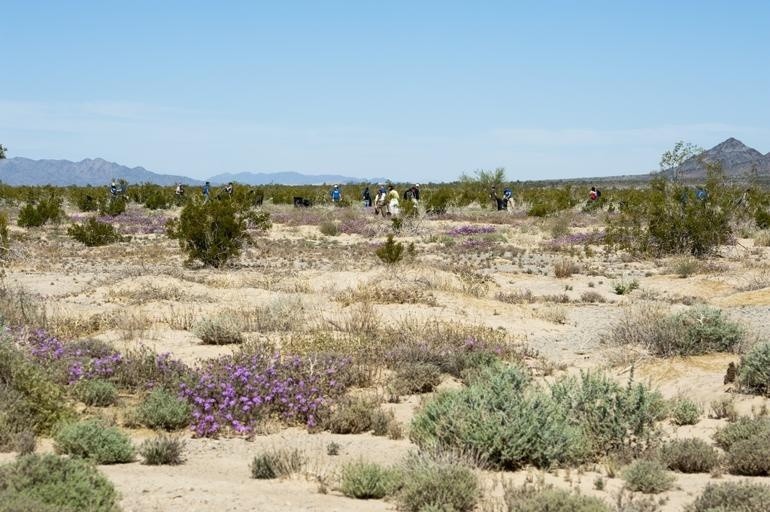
top-left (360, 180), bottom-right (423, 221)
top-left (489, 185), bottom-right (498, 209)
top-left (589, 186), bottom-right (597, 199)
top-left (226, 183), bottom-right (233, 201)
top-left (110, 178), bottom-right (116, 194)
top-left (202, 182), bottom-right (212, 207)
top-left (180, 185), bottom-right (184, 195)
top-left (330, 184), bottom-right (342, 204)
top-left (502, 187), bottom-right (511, 201)
top-left (681, 186), bottom-right (753, 209)
top-left (175, 183), bottom-right (180, 194)
top-left (116, 179), bottom-right (126, 192)
